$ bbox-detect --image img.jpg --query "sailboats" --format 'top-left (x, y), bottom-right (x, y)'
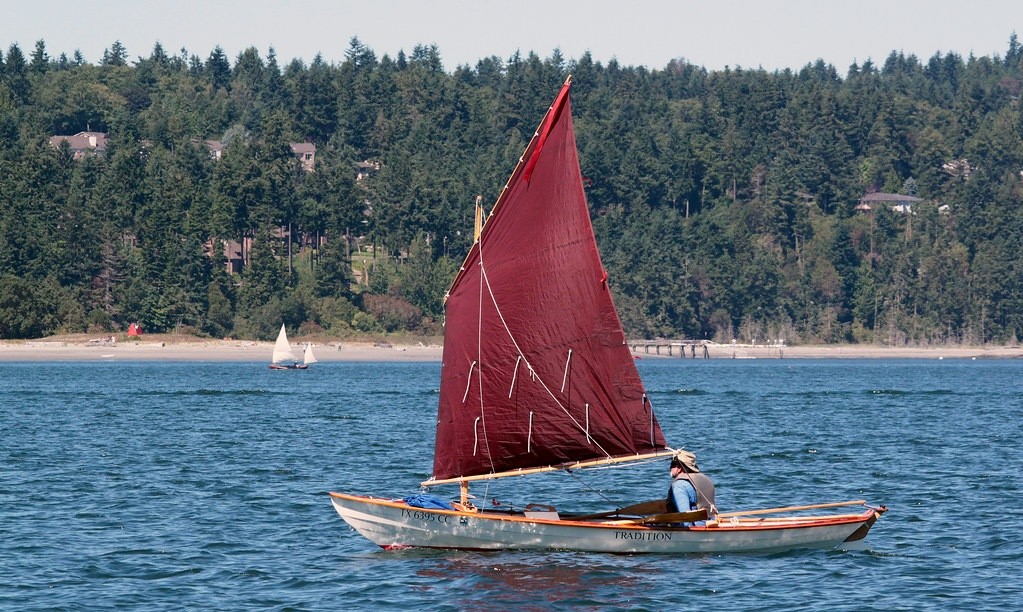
top-left (295, 341), bottom-right (318, 369)
top-left (329, 75), bottom-right (889, 554)
top-left (269, 323), bottom-right (298, 369)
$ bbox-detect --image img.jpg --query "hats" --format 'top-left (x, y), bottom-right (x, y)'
top-left (672, 449), bottom-right (699, 472)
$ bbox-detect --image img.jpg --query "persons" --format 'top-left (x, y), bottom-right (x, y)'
top-left (666, 451), bottom-right (715, 527)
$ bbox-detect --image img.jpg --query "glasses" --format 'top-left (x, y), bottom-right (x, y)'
top-left (671, 460), bottom-right (679, 468)
